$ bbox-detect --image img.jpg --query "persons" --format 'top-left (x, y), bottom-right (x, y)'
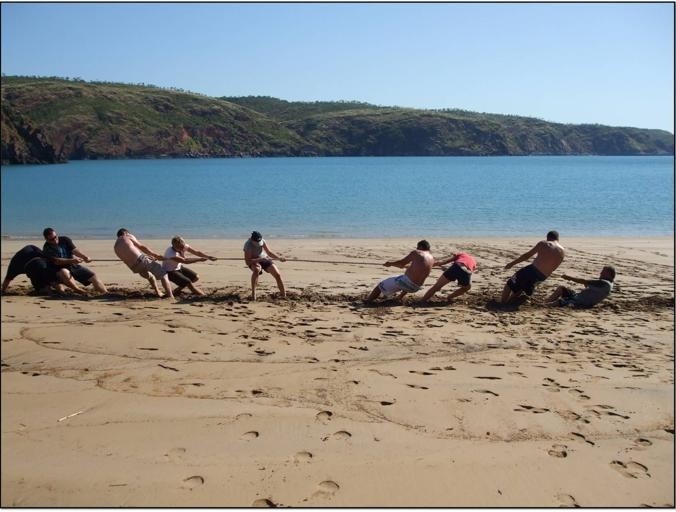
top-left (498, 231), bottom-right (564, 304)
top-left (363, 240), bottom-right (434, 301)
top-left (243, 231), bottom-right (287, 301)
top-left (114, 227), bottom-right (177, 299)
top-left (43, 227), bottom-right (109, 294)
top-left (532, 266), bottom-right (616, 310)
top-left (3, 245), bottom-right (64, 295)
top-left (417, 251), bottom-right (476, 303)
top-left (162, 235), bottom-right (216, 297)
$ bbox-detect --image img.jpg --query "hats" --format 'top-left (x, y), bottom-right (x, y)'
top-left (251, 231), bottom-right (263, 248)
